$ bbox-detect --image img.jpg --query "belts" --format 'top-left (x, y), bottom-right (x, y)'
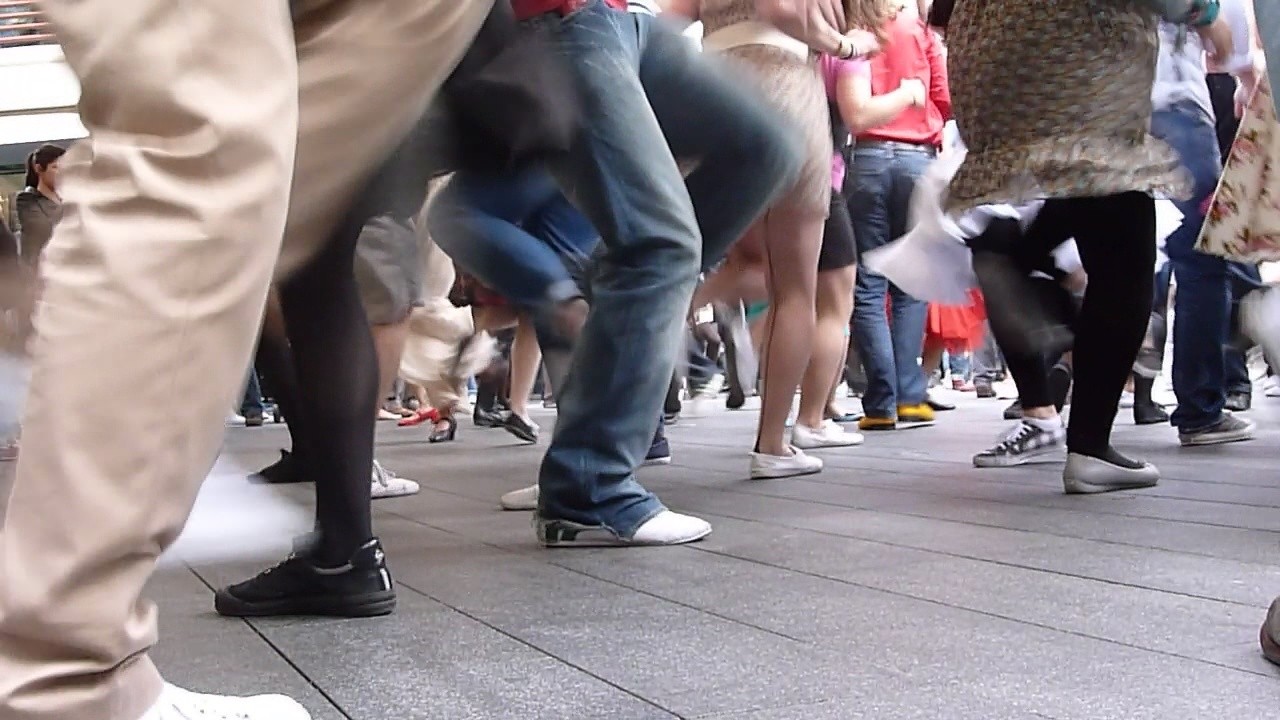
top-left (701, 20), bottom-right (808, 62)
top-left (856, 138), bottom-right (933, 153)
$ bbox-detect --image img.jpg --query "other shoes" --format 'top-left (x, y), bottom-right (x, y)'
top-left (850, 365), bottom-right (996, 430)
top-left (1178, 409), bottom-right (1256, 444)
top-left (833, 412), bottom-right (865, 421)
top-left (1223, 392), bottom-right (1253, 410)
top-left (727, 388), bottom-right (746, 410)
top-left (230, 360), bottom-right (541, 444)
top-left (1118, 391), bottom-right (1134, 407)
top-left (642, 436), bottom-right (671, 467)
top-left (690, 374), bottom-right (725, 412)
top-left (662, 411), bottom-right (681, 424)
top-left (1133, 399), bottom-right (1170, 424)
top-left (136, 682), bottom-right (310, 720)
top-left (1260, 595), bottom-right (1280, 666)
top-left (1004, 401), bottom-right (1025, 419)
top-left (1265, 375), bottom-right (1280, 396)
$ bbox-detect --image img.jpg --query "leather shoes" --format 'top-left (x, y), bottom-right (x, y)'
top-left (1062, 450), bottom-right (1159, 494)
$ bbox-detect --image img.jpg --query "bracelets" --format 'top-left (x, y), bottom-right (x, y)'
top-left (834, 40), bottom-right (842, 57)
top-left (840, 43), bottom-right (854, 60)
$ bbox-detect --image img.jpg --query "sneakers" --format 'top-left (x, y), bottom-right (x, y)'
top-left (536, 503), bottom-right (712, 547)
top-left (972, 419), bottom-right (1067, 467)
top-left (215, 536), bottom-right (397, 618)
top-left (369, 459), bottom-right (419, 498)
top-left (792, 418), bottom-right (865, 448)
top-left (500, 482), bottom-right (539, 510)
top-left (246, 448), bottom-right (313, 484)
top-left (749, 444), bottom-right (823, 478)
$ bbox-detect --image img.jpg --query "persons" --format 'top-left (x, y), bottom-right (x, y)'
top-left (636, 0), bottom-right (1089, 478)
top-left (214, 101), bottom-right (604, 618)
top-left (0, 143), bottom-right (66, 460)
top-left (1120, 0), bottom-right (1280, 445)
top-left (515, 0), bottom-right (790, 546)
top-left (0, 1), bottom-right (498, 720)
top-left (927, 0), bottom-right (1232, 493)
